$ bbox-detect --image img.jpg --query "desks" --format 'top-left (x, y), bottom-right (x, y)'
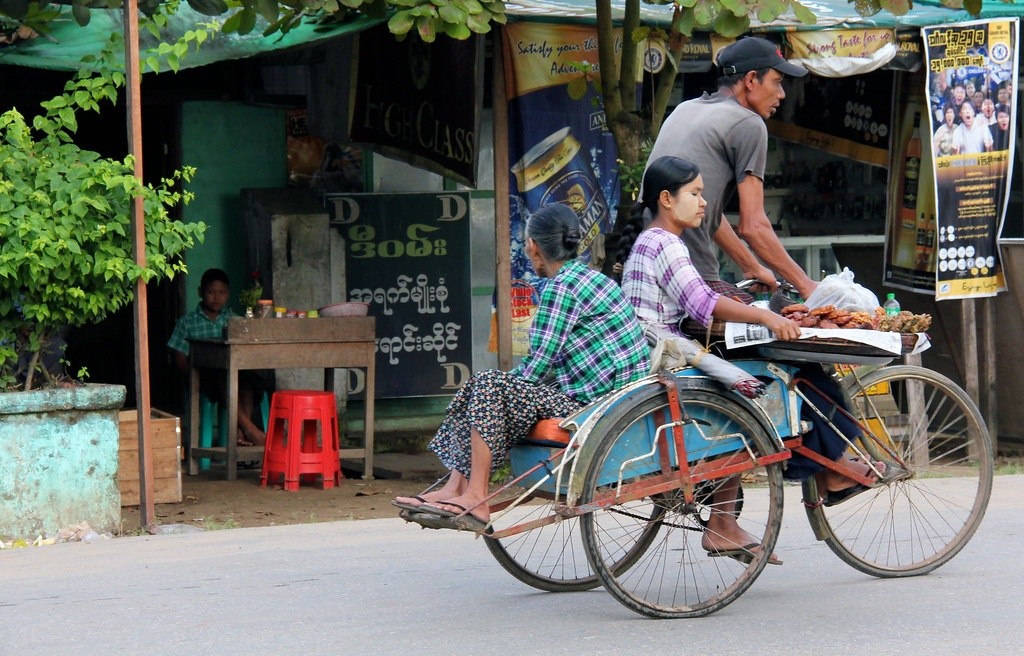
top-left (181, 315), bottom-right (377, 485)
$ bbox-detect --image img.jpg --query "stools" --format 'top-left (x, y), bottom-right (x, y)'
top-left (258, 388), bottom-right (343, 493)
top-left (199, 388), bottom-right (271, 472)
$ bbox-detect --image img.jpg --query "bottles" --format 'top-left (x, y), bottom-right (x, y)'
top-left (273, 306), bottom-right (286, 317)
top-left (257, 298), bottom-right (273, 317)
top-left (884, 293), bottom-right (900, 316)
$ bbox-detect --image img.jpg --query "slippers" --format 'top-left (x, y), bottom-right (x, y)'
top-left (418, 500), bottom-right (493, 530)
top-left (821, 454), bottom-right (893, 508)
top-left (706, 542), bottom-right (785, 566)
top-left (390, 494), bottom-right (429, 514)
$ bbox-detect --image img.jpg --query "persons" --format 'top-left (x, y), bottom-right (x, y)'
top-left (637, 38), bottom-right (888, 508)
top-left (164, 269), bottom-right (270, 449)
top-left (612, 156), bottom-right (804, 564)
top-left (931, 67), bottom-right (1011, 156)
top-left (389, 201), bottom-right (650, 534)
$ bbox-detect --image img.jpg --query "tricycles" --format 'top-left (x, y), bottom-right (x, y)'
top-left (461, 274), bottom-right (992, 617)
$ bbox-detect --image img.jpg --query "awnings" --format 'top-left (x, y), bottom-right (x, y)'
top-left (1, 0), bottom-right (514, 533)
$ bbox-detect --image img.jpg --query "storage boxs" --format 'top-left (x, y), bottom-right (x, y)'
top-left (114, 407), bottom-right (184, 509)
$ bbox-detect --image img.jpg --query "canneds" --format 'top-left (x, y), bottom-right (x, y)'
top-left (510, 126), bottom-right (614, 263)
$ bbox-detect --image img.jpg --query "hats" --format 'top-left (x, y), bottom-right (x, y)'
top-left (715, 35), bottom-right (809, 78)
